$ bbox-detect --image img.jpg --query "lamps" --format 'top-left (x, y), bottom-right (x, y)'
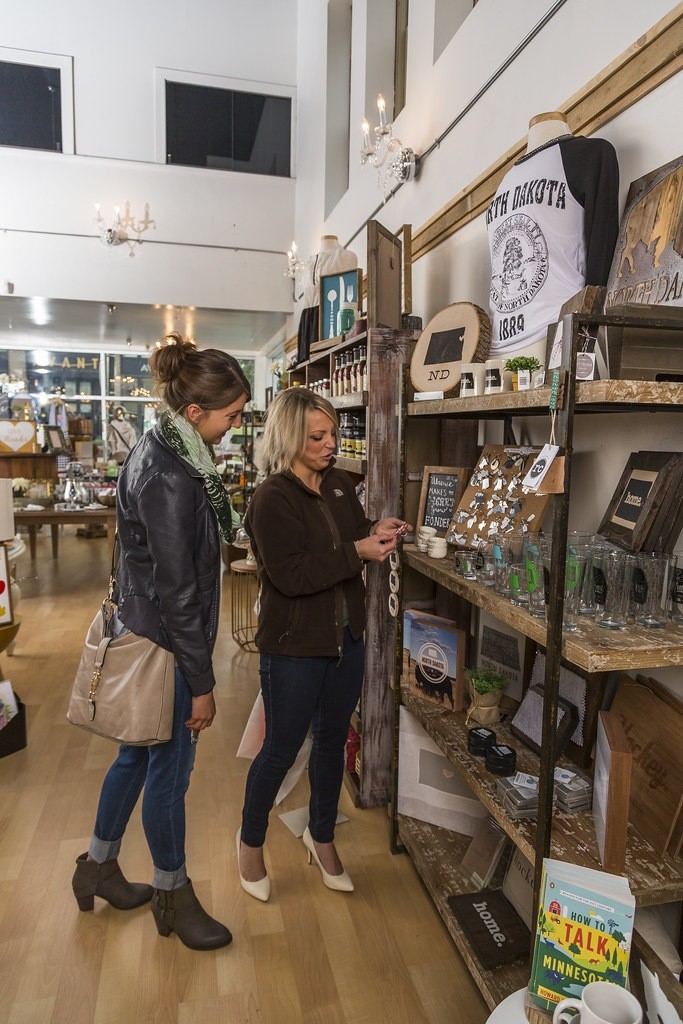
top-left (360, 93), bottom-right (418, 182)
top-left (93, 203), bottom-right (142, 246)
top-left (285, 239), bottom-right (303, 301)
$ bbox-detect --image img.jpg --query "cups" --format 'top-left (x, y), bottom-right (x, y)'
top-left (460, 361), bottom-right (485, 397)
top-left (416, 526), bottom-right (447, 558)
top-left (455, 531), bottom-right (683, 631)
top-left (484, 359), bottom-right (513, 395)
top-left (553, 981), bottom-right (643, 1024)
top-left (337, 412), bottom-right (366, 460)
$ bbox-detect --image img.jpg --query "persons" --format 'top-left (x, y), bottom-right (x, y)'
top-left (72, 332), bottom-right (250, 951)
top-left (297, 235), bottom-right (357, 365)
top-left (237, 387), bottom-right (413, 902)
top-left (487, 112), bottom-right (620, 394)
top-left (110, 407), bottom-right (137, 456)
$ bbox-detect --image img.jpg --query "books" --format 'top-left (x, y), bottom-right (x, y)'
top-left (458, 816), bottom-right (508, 889)
top-left (528, 859), bottom-right (636, 1016)
top-left (402, 609), bottom-right (465, 711)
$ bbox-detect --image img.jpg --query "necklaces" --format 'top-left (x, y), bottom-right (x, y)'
top-left (517, 134), bottom-right (573, 162)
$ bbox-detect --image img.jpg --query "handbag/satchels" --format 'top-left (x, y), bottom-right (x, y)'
top-left (66, 599), bottom-right (175, 745)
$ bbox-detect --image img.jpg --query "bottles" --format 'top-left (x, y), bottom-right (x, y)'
top-left (232, 472), bottom-right (244, 486)
top-left (336, 301), bottom-right (359, 335)
top-left (293, 346), bottom-right (368, 396)
top-left (339, 308), bottom-right (354, 333)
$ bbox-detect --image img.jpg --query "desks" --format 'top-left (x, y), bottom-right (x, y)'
top-left (231, 560), bottom-right (261, 654)
top-left (14, 501), bottom-right (117, 579)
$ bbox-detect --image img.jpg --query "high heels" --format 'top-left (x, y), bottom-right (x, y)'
top-left (72, 851), bottom-right (153, 910)
top-left (302, 826), bottom-right (355, 892)
top-left (150, 877), bottom-right (233, 951)
top-left (235, 826), bottom-right (270, 901)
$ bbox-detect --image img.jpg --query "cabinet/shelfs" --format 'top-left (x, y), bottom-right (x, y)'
top-left (242, 328), bottom-right (421, 807)
top-left (384, 313), bottom-right (683, 1024)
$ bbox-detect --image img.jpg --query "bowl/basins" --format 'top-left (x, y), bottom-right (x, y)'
top-left (95, 491), bottom-right (116, 507)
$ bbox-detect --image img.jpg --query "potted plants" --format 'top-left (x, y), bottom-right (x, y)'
top-left (503, 356), bottom-right (543, 391)
